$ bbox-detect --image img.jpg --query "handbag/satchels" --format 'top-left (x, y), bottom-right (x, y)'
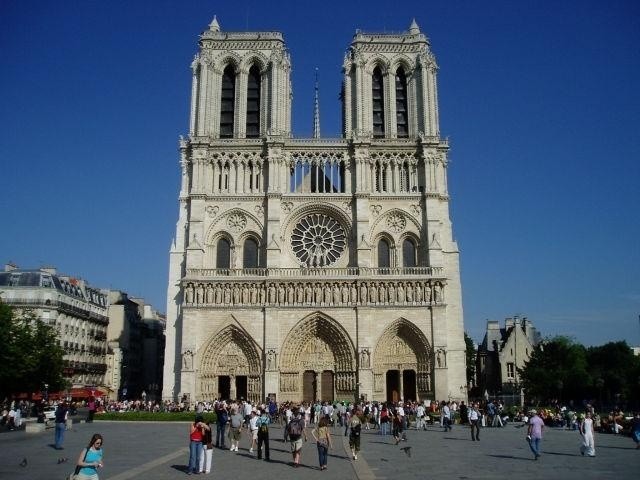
top-left (73, 447), bottom-right (89, 475)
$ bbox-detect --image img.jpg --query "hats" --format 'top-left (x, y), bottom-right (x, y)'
top-left (528, 410), bottom-right (537, 413)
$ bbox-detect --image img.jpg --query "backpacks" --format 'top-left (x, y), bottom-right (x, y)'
top-left (290, 419), bottom-right (303, 436)
top-left (259, 418), bottom-right (268, 433)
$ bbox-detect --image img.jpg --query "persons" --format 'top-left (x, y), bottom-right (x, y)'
top-left (69, 433), bottom-right (104, 480)
top-left (580, 411), bottom-right (596, 457)
top-left (1, 395), bottom-right (189, 430)
top-left (514, 398), bottom-right (638, 443)
top-left (346, 409), bottom-right (362, 459)
top-left (284, 411), bottom-right (307, 467)
top-left (527, 409), bottom-right (545, 460)
top-left (279, 398), bottom-right (508, 445)
top-left (187, 397), bottom-right (279, 476)
top-left (54, 403), bottom-right (69, 450)
top-left (311, 417), bottom-right (333, 470)
